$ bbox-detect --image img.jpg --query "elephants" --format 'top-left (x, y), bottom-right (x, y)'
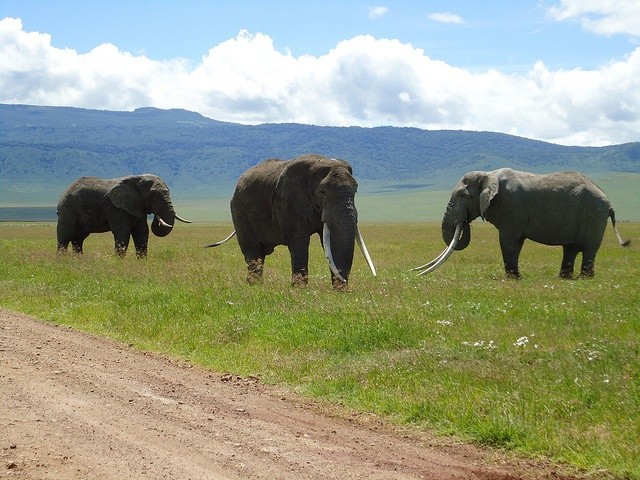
top-left (204, 154), bottom-right (377, 292)
top-left (54, 172), bottom-right (193, 260)
top-left (407, 166), bottom-right (631, 279)
